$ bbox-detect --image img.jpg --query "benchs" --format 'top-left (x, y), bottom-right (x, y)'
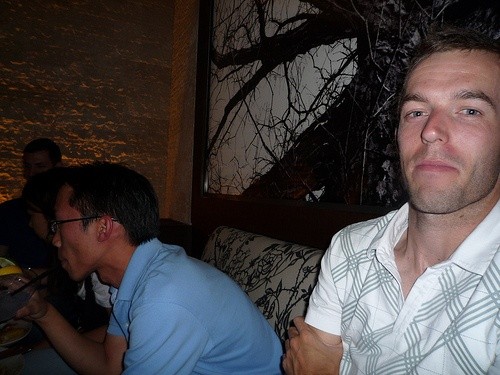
top-left (201, 226), bottom-right (324, 347)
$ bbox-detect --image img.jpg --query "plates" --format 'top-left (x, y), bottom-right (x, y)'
top-left (0, 319), bottom-right (32, 347)
top-left (0, 347), bottom-right (26, 375)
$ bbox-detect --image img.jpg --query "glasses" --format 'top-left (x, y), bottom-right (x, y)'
top-left (51, 215), bottom-right (118, 233)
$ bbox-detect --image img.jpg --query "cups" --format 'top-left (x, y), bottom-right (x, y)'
top-left (0, 268), bottom-right (41, 322)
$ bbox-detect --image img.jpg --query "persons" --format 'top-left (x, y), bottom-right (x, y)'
top-left (2, 159), bottom-right (285, 374)
top-left (21, 166), bottom-right (119, 334)
top-left (280, 23), bottom-right (500, 375)
top-left (1, 138), bottom-right (62, 271)
